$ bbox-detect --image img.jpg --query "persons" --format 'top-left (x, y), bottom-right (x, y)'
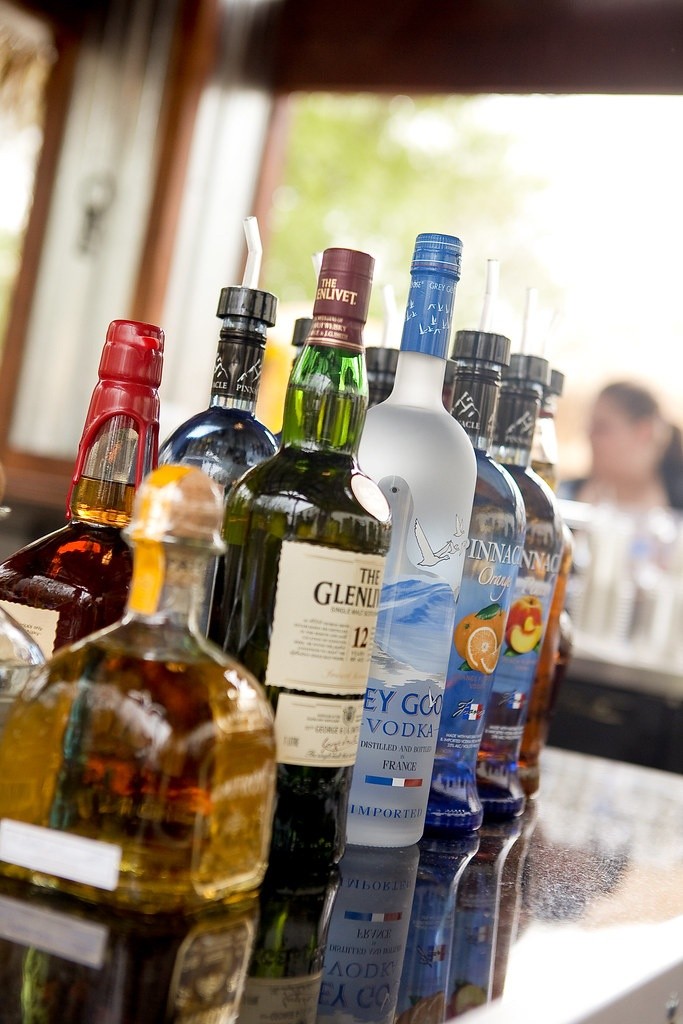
top-left (552, 379), bottom-right (682, 667)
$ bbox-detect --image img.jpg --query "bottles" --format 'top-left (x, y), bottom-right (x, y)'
top-left (1, 214), bottom-right (587, 915)
top-left (0, 801), bottom-right (540, 1024)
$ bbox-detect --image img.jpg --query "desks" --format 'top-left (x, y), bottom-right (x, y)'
top-left (442, 745), bottom-right (682, 1024)
